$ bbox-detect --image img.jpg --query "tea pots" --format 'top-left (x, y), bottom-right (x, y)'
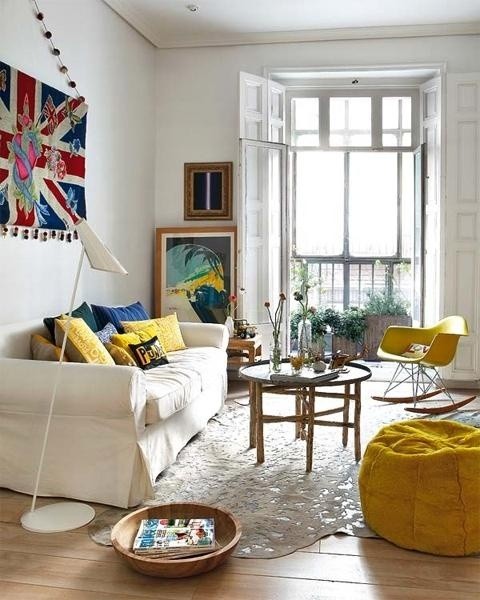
top-left (233, 318), bottom-right (250, 335)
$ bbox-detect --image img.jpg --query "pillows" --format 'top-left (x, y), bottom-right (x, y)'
top-left (401, 341), bottom-right (431, 361)
top-left (28, 300), bottom-right (190, 369)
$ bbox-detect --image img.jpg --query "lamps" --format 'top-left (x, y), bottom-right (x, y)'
top-left (18, 216), bottom-right (132, 534)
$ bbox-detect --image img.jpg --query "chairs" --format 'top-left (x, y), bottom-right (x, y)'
top-left (370, 313), bottom-right (478, 415)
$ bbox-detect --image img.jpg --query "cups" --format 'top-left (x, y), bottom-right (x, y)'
top-left (248, 327), bottom-right (257, 339)
top-left (288, 354), bottom-right (303, 375)
top-left (238, 327), bottom-right (247, 338)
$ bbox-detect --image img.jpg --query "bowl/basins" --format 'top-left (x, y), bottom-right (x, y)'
top-left (109, 499), bottom-right (243, 579)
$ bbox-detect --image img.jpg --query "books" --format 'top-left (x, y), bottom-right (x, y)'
top-left (132, 517), bottom-right (222, 560)
top-left (270, 366), bottom-right (339, 383)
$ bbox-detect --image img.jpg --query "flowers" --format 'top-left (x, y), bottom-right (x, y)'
top-left (265, 292), bottom-right (287, 373)
top-left (289, 240), bottom-right (323, 368)
top-left (225, 287), bottom-right (246, 317)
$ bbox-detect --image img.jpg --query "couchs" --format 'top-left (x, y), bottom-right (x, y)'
top-left (0, 306), bottom-right (234, 509)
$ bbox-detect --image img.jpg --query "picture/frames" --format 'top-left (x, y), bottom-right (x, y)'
top-left (154, 226), bottom-right (238, 324)
top-left (182, 161), bottom-right (233, 222)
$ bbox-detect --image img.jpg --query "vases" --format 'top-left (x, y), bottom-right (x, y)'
top-left (297, 316), bottom-right (314, 368)
top-left (224, 316), bottom-right (235, 339)
top-left (269, 344), bottom-right (282, 373)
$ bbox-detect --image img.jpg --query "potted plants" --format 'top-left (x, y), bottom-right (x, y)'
top-left (290, 256), bottom-right (413, 362)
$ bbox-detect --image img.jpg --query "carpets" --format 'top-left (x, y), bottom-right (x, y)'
top-left (83, 403), bottom-right (480, 560)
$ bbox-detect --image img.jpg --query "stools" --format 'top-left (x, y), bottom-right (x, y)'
top-left (357, 419), bottom-right (480, 558)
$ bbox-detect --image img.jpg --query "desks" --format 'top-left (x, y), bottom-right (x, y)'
top-left (226, 335), bottom-right (263, 396)
